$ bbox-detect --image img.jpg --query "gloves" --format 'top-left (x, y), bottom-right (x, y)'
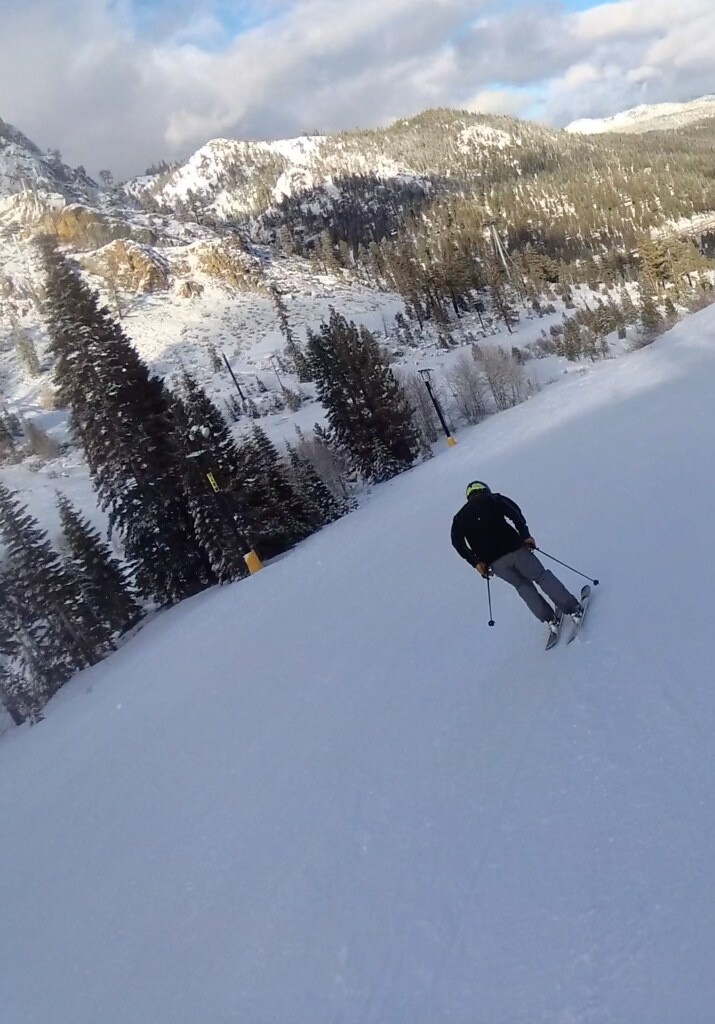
top-left (476, 563), bottom-right (494, 580)
top-left (523, 537), bottom-right (536, 553)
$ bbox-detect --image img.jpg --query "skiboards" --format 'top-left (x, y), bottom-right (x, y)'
top-left (545, 584), bottom-right (592, 650)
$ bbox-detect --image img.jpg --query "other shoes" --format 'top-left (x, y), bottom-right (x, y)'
top-left (547, 616), bottom-right (560, 631)
top-left (570, 607), bottom-right (584, 622)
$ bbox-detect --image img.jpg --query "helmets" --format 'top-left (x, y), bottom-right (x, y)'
top-left (466, 482), bottom-right (490, 499)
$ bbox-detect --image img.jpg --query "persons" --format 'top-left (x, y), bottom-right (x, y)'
top-left (451, 481), bottom-right (585, 634)
